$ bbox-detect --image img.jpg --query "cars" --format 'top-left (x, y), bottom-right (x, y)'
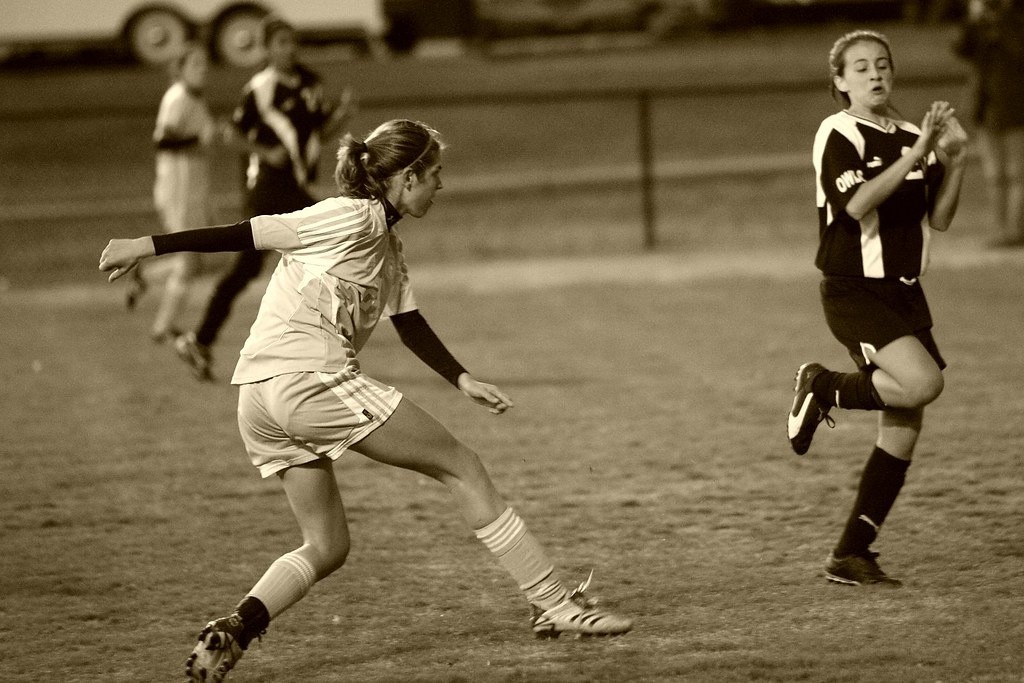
top-left (1, 0), bottom-right (389, 67)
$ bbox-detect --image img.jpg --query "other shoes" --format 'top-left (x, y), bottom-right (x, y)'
top-left (123, 261), bottom-right (148, 312)
top-left (148, 320), bottom-right (183, 346)
top-left (173, 330), bottom-right (216, 383)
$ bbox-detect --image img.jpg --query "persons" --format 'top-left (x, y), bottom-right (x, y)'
top-left (952, 1), bottom-right (1024, 246)
top-left (787, 29), bottom-right (969, 587)
top-left (99, 119), bottom-right (634, 683)
top-left (172, 18), bottom-right (357, 383)
top-left (126, 44), bottom-right (231, 342)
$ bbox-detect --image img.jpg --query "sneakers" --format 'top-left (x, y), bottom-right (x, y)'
top-left (823, 548), bottom-right (906, 593)
top-left (785, 362), bottom-right (835, 455)
top-left (185, 610), bottom-right (268, 683)
top-left (530, 568), bottom-right (634, 638)
